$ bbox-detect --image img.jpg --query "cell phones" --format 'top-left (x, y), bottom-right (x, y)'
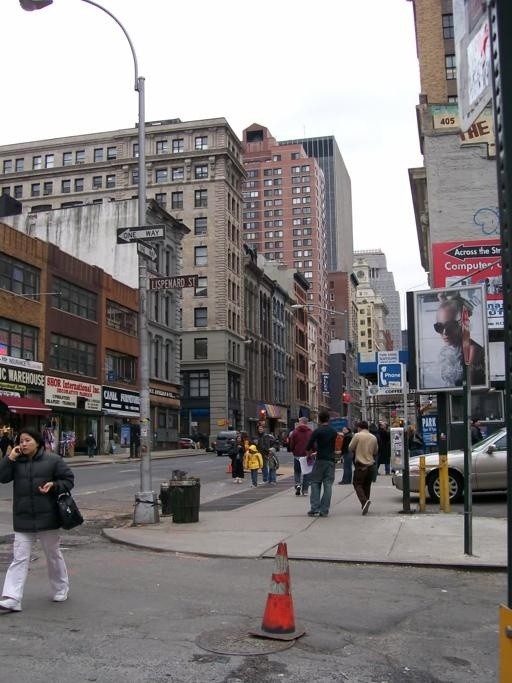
top-left (18, 448), bottom-right (23, 455)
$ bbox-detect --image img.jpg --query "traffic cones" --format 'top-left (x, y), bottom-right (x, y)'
top-left (250, 538), bottom-right (308, 643)
top-left (225, 462), bottom-right (232, 473)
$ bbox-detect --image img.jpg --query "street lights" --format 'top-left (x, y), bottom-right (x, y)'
top-left (290, 303), bottom-right (353, 427)
top-left (18, 0), bottom-right (163, 523)
top-left (222, 338), bottom-right (253, 430)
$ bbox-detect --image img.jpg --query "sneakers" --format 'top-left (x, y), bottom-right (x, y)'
top-left (52, 584), bottom-right (71, 603)
top-left (0, 598), bottom-right (23, 611)
top-left (293, 479), bottom-right (373, 518)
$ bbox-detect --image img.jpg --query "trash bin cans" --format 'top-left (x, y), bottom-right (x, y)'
top-left (158, 470), bottom-right (201, 523)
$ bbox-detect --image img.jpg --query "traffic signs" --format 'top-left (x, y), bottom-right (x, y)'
top-left (148, 274), bottom-right (199, 290)
top-left (116, 226), bottom-right (166, 245)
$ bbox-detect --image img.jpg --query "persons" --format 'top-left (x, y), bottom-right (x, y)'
top-left (471, 418), bottom-right (484, 443)
top-left (228, 409), bottom-right (416, 521)
top-left (427, 297), bottom-right (485, 384)
top-left (84, 432), bottom-right (96, 456)
top-left (0, 425), bottom-right (75, 612)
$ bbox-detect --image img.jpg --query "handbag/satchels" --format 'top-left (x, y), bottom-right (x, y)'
top-left (54, 483), bottom-right (83, 532)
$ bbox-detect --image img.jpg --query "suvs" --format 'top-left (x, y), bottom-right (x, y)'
top-left (214, 430), bottom-right (240, 456)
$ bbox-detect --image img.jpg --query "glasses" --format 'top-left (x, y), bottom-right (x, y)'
top-left (432, 318), bottom-right (462, 335)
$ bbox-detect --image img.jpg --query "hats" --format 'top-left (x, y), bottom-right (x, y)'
top-left (14, 428), bottom-right (46, 444)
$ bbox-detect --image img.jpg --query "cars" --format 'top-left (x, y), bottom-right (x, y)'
top-left (392, 424), bottom-right (507, 503)
top-left (253, 427), bottom-right (293, 451)
top-left (179, 437), bottom-right (196, 449)
